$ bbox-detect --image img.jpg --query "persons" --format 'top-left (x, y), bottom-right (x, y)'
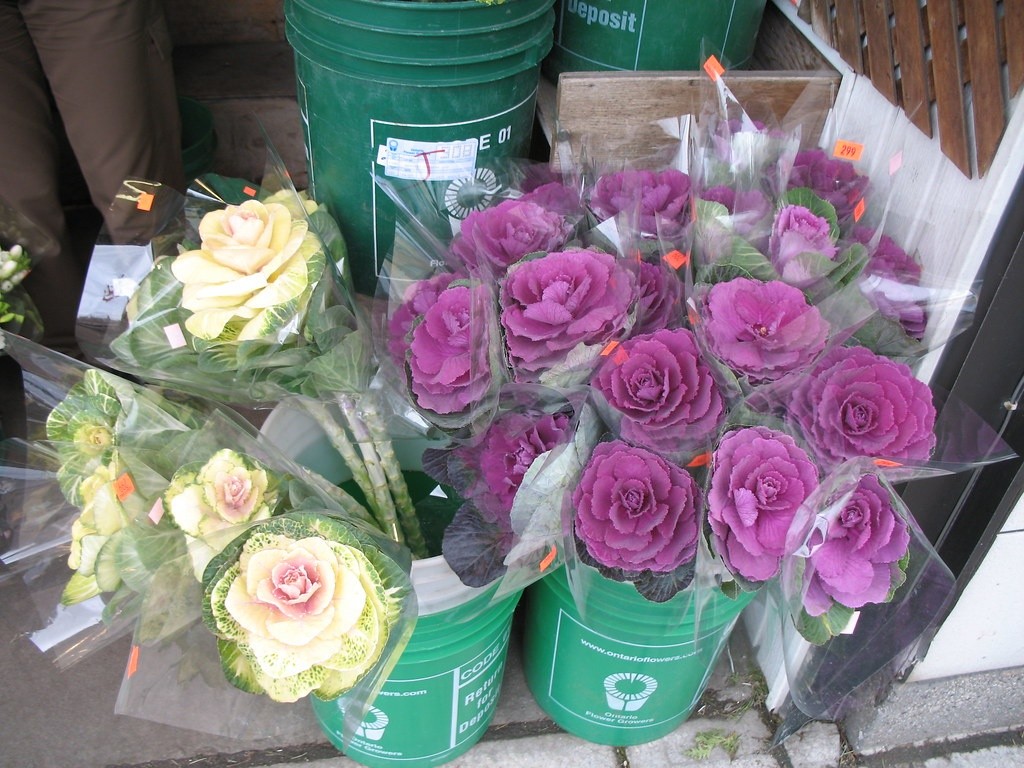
top-left (1, 1), bottom-right (197, 402)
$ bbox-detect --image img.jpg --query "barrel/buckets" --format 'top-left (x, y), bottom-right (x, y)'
top-left (282, 0), bottom-right (556, 298)
top-left (302, 558), bottom-right (528, 768)
top-left (527, 544), bottom-right (764, 747)
top-left (544, 0), bottom-right (767, 88)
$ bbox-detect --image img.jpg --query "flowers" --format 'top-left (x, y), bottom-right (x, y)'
top-left (46, 119), bottom-right (938, 701)
top-left (1, 244), bottom-right (33, 323)
